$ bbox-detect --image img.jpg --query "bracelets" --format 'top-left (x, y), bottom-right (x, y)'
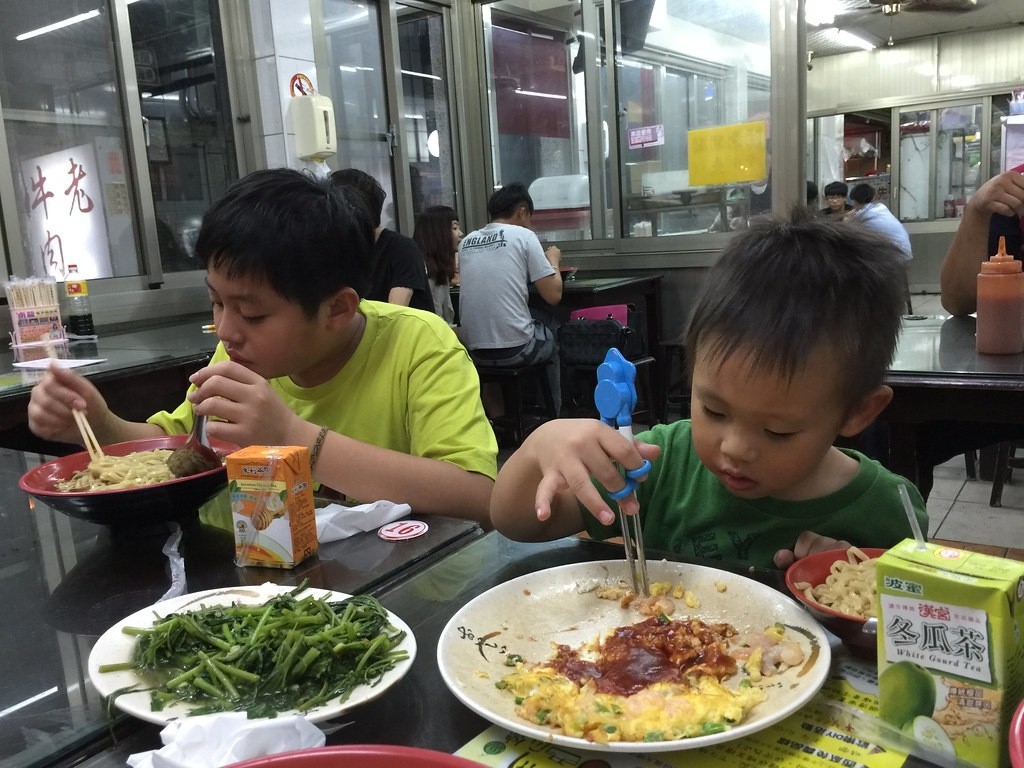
top-left (552, 265), bottom-right (559, 270)
top-left (310, 426), bottom-right (330, 474)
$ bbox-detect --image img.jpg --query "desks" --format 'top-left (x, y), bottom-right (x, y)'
top-left (0, 447), bottom-right (484, 768)
top-left (450, 273), bottom-right (666, 427)
top-left (837, 315), bottom-right (1024, 489)
top-left (0, 316), bottom-right (220, 403)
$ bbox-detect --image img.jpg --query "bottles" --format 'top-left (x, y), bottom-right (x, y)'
top-left (955, 197), bottom-right (965, 217)
top-left (63, 265), bottom-right (95, 336)
top-left (886, 164), bottom-right (890, 173)
top-left (976, 236), bottom-right (1023, 354)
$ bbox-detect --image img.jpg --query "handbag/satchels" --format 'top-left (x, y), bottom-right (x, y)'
top-left (556, 302), bottom-right (646, 366)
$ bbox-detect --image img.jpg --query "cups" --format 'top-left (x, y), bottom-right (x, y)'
top-left (944, 200), bottom-right (954, 218)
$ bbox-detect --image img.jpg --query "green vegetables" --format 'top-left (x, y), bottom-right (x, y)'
top-left (97, 575), bottom-right (410, 745)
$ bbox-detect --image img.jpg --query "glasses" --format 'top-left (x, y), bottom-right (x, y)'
top-left (826, 195), bottom-right (846, 203)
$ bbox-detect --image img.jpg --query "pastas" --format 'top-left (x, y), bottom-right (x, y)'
top-left (793, 547), bottom-right (883, 621)
top-left (53, 444), bottom-right (185, 493)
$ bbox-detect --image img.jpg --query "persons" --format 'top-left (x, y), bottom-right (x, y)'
top-left (116, 217), bottom-right (202, 273)
top-left (483, 197), bottom-right (930, 571)
top-left (807, 180), bottom-right (818, 207)
top-left (329, 165), bottom-right (436, 314)
top-left (459, 182), bottom-right (565, 428)
top-left (28, 166), bottom-right (499, 529)
top-left (917, 168), bottom-right (1024, 506)
top-left (707, 113), bottom-right (772, 230)
top-left (818, 180), bottom-right (852, 222)
top-left (847, 183), bottom-right (914, 263)
top-left (411, 204), bottom-right (464, 327)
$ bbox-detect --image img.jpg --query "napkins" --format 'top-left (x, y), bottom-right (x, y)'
top-left (315, 499), bottom-right (410, 542)
top-left (125, 711), bottom-right (327, 768)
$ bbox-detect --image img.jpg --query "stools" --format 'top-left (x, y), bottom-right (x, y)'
top-left (473, 362), bottom-right (555, 449)
top-left (658, 335), bottom-right (690, 424)
top-left (572, 355), bottom-right (658, 432)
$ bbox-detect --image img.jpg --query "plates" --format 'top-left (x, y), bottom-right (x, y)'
top-left (437, 558), bottom-right (831, 752)
top-left (87, 586), bottom-right (417, 726)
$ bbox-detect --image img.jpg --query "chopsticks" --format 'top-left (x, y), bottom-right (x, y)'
top-left (2, 275), bottom-right (56, 306)
top-left (43, 336), bottom-right (102, 460)
top-left (593, 348), bottom-right (651, 598)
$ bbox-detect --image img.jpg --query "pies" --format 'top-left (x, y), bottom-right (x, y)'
top-left (495, 613), bottom-right (783, 743)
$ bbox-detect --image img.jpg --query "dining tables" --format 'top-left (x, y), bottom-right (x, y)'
top-left (59, 526), bottom-right (940, 768)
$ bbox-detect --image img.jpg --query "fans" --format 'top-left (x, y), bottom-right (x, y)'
top-left (810, 0), bottom-right (985, 33)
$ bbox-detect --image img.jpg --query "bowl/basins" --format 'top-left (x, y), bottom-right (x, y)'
top-left (18, 434), bottom-right (243, 528)
top-left (1008, 697), bottom-right (1024, 767)
top-left (560, 267), bottom-right (579, 281)
top-left (220, 745), bottom-right (491, 768)
top-left (784, 548), bottom-right (890, 656)
